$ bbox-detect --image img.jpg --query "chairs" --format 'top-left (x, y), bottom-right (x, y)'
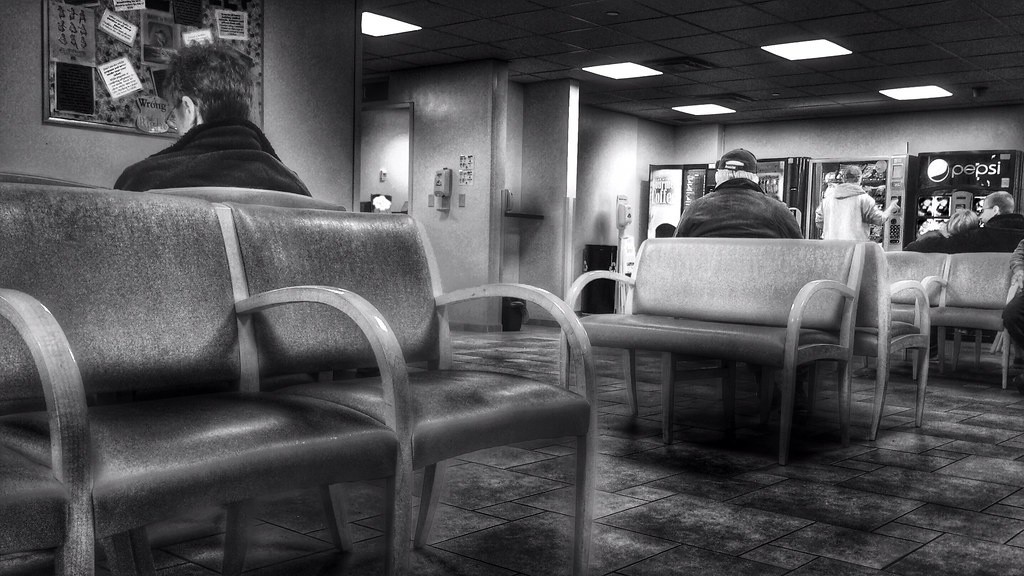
top-left (885, 249), bottom-right (1015, 388)
top-left (0, 183), bottom-right (599, 576)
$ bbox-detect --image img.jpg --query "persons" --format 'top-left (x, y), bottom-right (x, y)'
top-left (114, 43), bottom-right (312, 197)
top-left (903, 191), bottom-right (1024, 254)
top-left (815, 165), bottom-right (900, 242)
top-left (1001, 239), bottom-right (1024, 351)
top-left (673, 148), bottom-right (808, 418)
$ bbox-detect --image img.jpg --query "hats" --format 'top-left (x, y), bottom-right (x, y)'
top-left (718, 148), bottom-right (758, 173)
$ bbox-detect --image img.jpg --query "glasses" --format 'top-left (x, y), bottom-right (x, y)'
top-left (978, 206), bottom-right (993, 213)
top-left (165, 99), bottom-right (198, 131)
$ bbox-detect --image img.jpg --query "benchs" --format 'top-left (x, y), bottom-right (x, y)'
top-left (558, 234), bottom-right (864, 468)
top-left (859, 240), bottom-right (931, 440)
